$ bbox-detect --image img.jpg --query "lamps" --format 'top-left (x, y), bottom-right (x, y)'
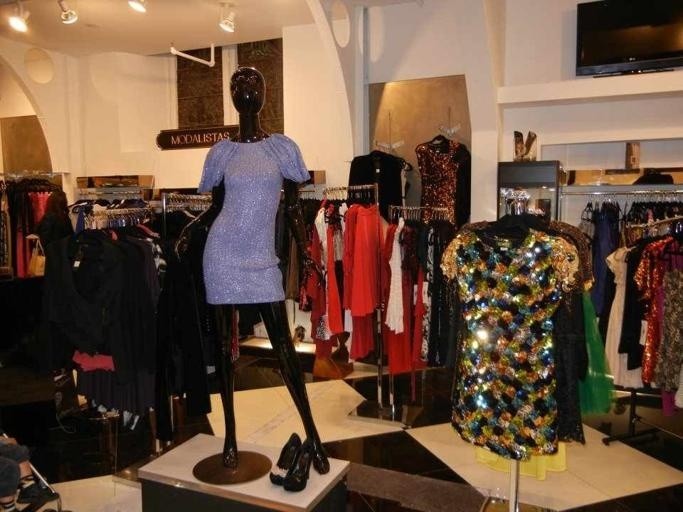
top-left (51, 0), bottom-right (80, 25)
top-left (4, 0), bottom-right (33, 36)
top-left (217, 2), bottom-right (236, 34)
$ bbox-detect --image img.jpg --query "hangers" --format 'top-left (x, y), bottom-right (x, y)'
top-left (275, 124), bottom-right (468, 238)
top-left (459, 178), bottom-right (681, 258)
top-left (0, 167), bottom-right (213, 246)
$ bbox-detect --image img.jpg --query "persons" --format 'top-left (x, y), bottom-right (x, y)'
top-left (0, 435), bottom-right (60, 512)
top-left (175, 65), bottom-right (331, 474)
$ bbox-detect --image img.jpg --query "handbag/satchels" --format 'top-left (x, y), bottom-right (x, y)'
top-left (29, 239), bottom-right (45, 276)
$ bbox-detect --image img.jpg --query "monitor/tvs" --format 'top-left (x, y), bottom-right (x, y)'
top-left (575, 0), bottom-right (683, 78)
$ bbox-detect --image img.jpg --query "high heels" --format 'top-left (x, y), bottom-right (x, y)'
top-left (270, 433), bottom-right (302, 485)
top-left (283, 438), bottom-right (314, 492)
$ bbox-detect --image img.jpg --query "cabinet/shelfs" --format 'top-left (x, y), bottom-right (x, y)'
top-left (74, 174), bottom-right (156, 192)
top-left (490, 66), bottom-right (683, 193)
